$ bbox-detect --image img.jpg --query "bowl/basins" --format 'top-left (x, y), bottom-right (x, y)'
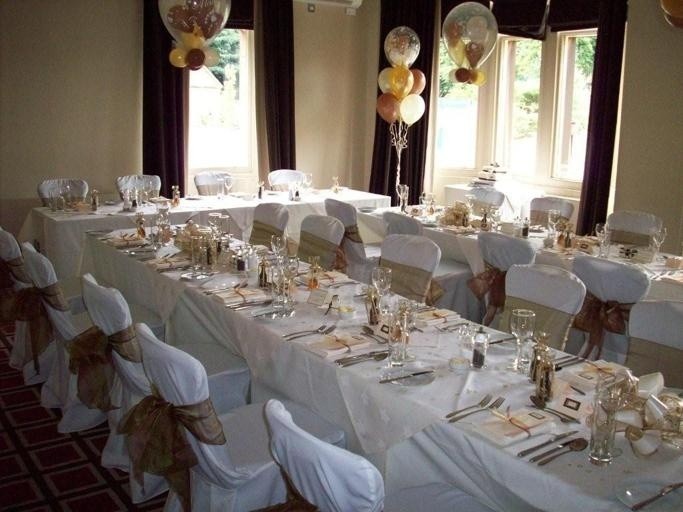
top-left (338, 309), bottom-right (357, 320)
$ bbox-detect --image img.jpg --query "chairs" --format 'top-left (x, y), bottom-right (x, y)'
top-left (296, 215), bottom-right (342, 270)
top-left (135, 328), bottom-right (346, 511)
top-left (1, 229), bottom-right (85, 368)
top-left (264, 396), bottom-right (490, 511)
top-left (249, 202), bottom-right (290, 247)
top-left (26, 243), bottom-right (160, 418)
top-left (78, 273), bottom-right (249, 473)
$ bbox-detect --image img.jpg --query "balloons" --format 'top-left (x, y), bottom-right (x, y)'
top-left (378, 26), bottom-right (428, 128)
top-left (442, 2), bottom-right (498, 87)
top-left (158, 0), bottom-right (231, 70)
top-left (663, 13), bottom-right (683, 27)
top-left (660, 0), bottom-right (683, 18)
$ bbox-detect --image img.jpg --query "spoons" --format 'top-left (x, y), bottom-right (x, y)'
top-left (537, 438), bottom-right (586, 469)
top-left (554, 355), bottom-right (584, 371)
top-left (233, 300), bottom-right (272, 313)
top-left (529, 394), bottom-right (580, 425)
top-left (337, 352), bottom-right (387, 369)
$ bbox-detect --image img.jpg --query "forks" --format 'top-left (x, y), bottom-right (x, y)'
top-left (202, 283), bottom-right (249, 297)
top-left (281, 323), bottom-right (336, 343)
top-left (527, 406), bottom-right (568, 423)
top-left (432, 322), bottom-right (469, 332)
top-left (323, 281), bottom-right (358, 290)
top-left (444, 393), bottom-right (505, 426)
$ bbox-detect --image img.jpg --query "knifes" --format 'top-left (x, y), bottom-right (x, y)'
top-left (630, 481), bottom-right (682, 510)
top-left (488, 337), bottom-right (517, 345)
top-left (378, 370), bottom-right (432, 385)
top-left (513, 430), bottom-right (580, 463)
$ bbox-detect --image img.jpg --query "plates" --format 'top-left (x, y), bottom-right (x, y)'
top-left (612, 475), bottom-right (682, 512)
top-left (379, 366), bottom-right (435, 387)
top-left (250, 307), bottom-right (294, 322)
top-left (486, 330), bottom-right (518, 352)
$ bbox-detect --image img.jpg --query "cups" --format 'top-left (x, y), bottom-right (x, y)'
top-left (189, 210), bottom-right (286, 277)
top-left (588, 367), bottom-right (616, 466)
top-left (387, 313), bottom-right (406, 368)
top-left (216, 179), bottom-right (223, 200)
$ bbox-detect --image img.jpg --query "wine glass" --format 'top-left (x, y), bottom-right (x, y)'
top-left (395, 182), bottom-right (666, 259)
top-left (265, 235), bottom-right (298, 307)
top-left (507, 308), bottom-right (535, 369)
top-left (303, 172), bottom-right (314, 193)
top-left (224, 176), bottom-right (233, 197)
top-left (371, 266), bottom-right (393, 308)
top-left (596, 369), bottom-right (632, 460)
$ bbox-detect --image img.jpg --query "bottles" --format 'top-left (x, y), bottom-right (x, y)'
top-left (256, 184), bottom-right (300, 201)
top-left (330, 294), bottom-right (340, 317)
top-left (45, 185), bottom-right (180, 243)
top-left (460, 210), bottom-right (529, 239)
top-left (306, 255), bottom-right (321, 291)
top-left (470, 327), bottom-right (491, 370)
